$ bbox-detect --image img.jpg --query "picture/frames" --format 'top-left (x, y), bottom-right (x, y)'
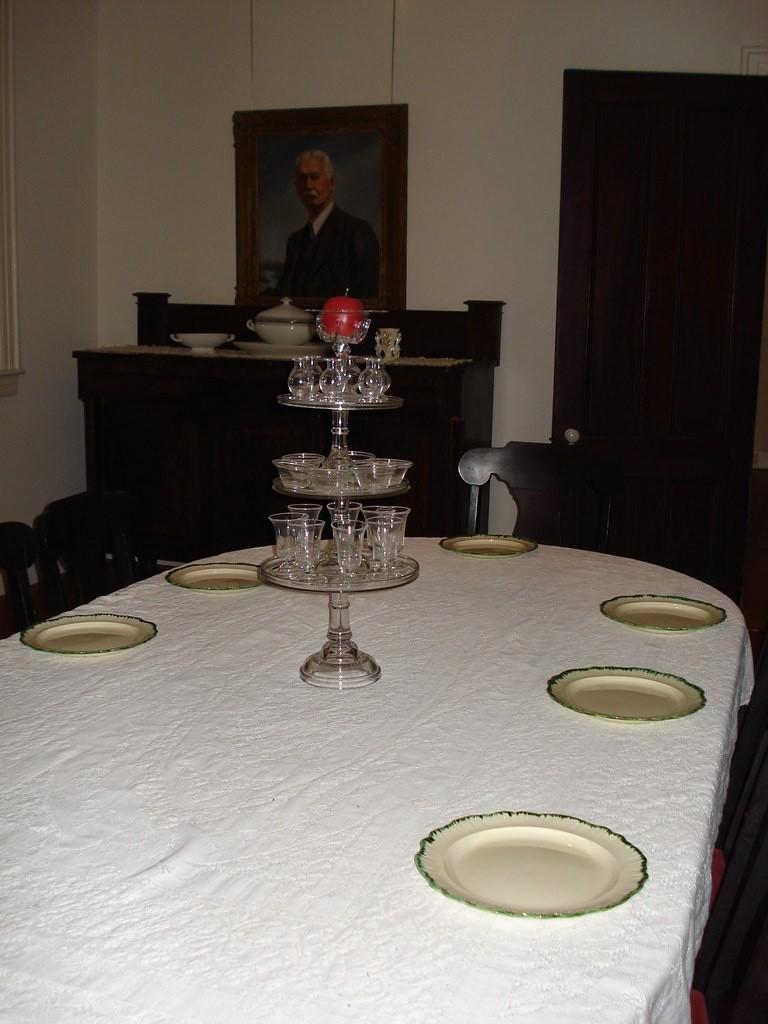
top-left (231, 101), bottom-right (409, 310)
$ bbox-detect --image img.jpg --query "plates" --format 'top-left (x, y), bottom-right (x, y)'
top-left (414, 811), bottom-right (648, 919)
top-left (438, 534), bottom-right (539, 560)
top-left (600, 594), bottom-right (727, 635)
top-left (233, 342), bottom-right (329, 357)
top-left (19, 614), bottom-right (159, 660)
top-left (166, 563), bottom-right (262, 598)
top-left (546, 666), bottom-right (707, 725)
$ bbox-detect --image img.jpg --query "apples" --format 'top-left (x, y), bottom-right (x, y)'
top-left (320, 288), bottom-right (364, 337)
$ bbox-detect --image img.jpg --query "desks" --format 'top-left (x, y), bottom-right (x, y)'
top-left (0, 535), bottom-right (758, 1024)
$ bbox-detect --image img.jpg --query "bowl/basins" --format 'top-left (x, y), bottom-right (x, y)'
top-left (168, 333), bottom-right (234, 353)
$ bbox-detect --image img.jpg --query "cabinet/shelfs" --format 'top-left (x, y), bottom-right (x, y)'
top-left (68, 299), bottom-right (503, 539)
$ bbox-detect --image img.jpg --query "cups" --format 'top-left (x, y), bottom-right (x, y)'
top-left (273, 450), bottom-right (414, 493)
top-left (287, 355), bottom-right (391, 403)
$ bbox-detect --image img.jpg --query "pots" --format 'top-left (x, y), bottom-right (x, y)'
top-left (246, 296), bottom-right (317, 346)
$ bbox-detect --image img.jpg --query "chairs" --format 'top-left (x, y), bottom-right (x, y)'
top-left (455, 438), bottom-right (622, 554)
top-left (0, 522), bottom-right (40, 639)
top-left (35, 491), bottom-right (157, 614)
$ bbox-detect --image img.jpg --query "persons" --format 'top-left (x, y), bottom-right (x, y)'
top-left (275, 149), bottom-right (379, 298)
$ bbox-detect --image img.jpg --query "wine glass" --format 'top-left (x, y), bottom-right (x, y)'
top-left (268, 502), bottom-right (410, 585)
